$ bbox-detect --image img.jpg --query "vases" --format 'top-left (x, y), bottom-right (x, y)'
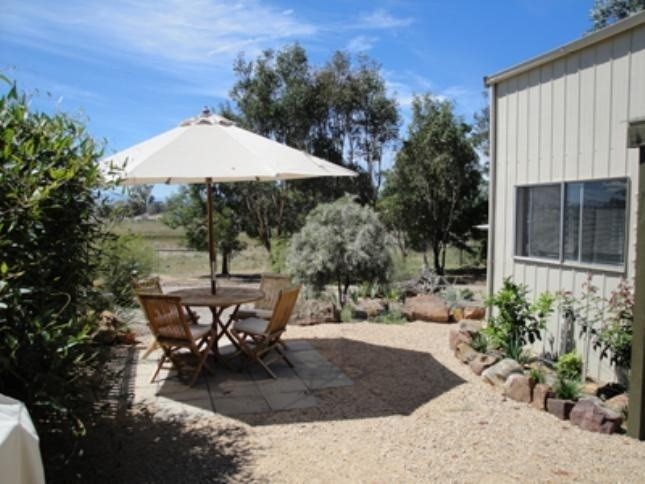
top-left (615, 365), bottom-right (632, 393)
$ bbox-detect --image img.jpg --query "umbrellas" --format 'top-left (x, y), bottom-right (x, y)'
top-left (88, 107), bottom-right (360, 373)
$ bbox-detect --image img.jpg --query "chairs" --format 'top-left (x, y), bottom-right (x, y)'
top-left (130, 272), bottom-right (302, 389)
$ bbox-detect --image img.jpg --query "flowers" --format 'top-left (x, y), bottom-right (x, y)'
top-left (557, 272), bottom-right (636, 365)
